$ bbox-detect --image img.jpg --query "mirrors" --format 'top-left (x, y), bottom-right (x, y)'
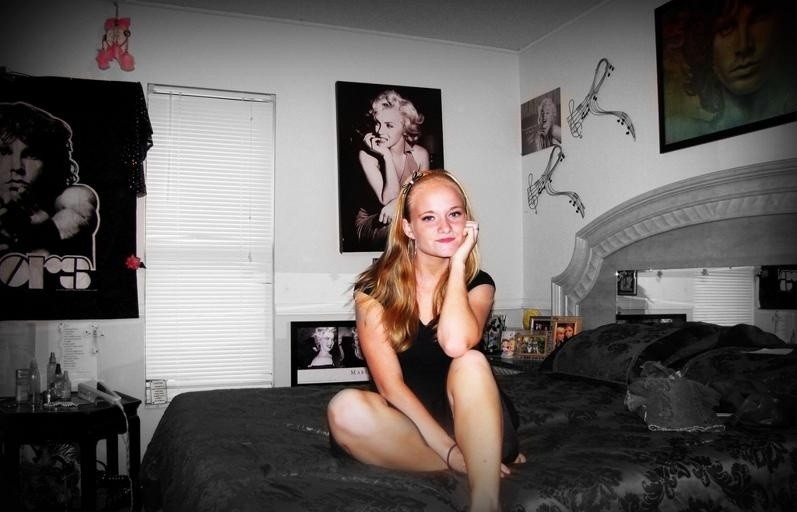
top-left (616, 263), bottom-right (796, 353)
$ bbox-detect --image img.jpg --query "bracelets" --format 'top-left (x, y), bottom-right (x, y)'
top-left (446, 443), bottom-right (458, 469)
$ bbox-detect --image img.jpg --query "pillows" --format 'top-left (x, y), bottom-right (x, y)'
top-left (539, 322), bottom-right (796, 428)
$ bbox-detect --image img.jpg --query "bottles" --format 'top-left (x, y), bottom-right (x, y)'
top-left (15, 352), bottom-right (72, 405)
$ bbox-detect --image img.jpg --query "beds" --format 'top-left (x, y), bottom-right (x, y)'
top-left (150, 157), bottom-right (796, 510)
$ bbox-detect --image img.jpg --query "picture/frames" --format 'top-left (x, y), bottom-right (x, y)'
top-left (291, 320), bottom-right (373, 388)
top-left (516, 315), bottom-right (582, 360)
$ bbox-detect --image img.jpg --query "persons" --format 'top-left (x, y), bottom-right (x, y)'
top-left (327, 169), bottom-right (526, 511)
top-left (502, 323), bottom-right (574, 354)
top-left (0, 101), bottom-right (100, 290)
top-left (307, 326), bottom-right (336, 367)
top-left (532, 98), bottom-right (560, 151)
top-left (352, 92), bottom-right (433, 248)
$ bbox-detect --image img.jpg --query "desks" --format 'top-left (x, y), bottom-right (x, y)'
top-left (0, 391), bottom-right (141, 511)
